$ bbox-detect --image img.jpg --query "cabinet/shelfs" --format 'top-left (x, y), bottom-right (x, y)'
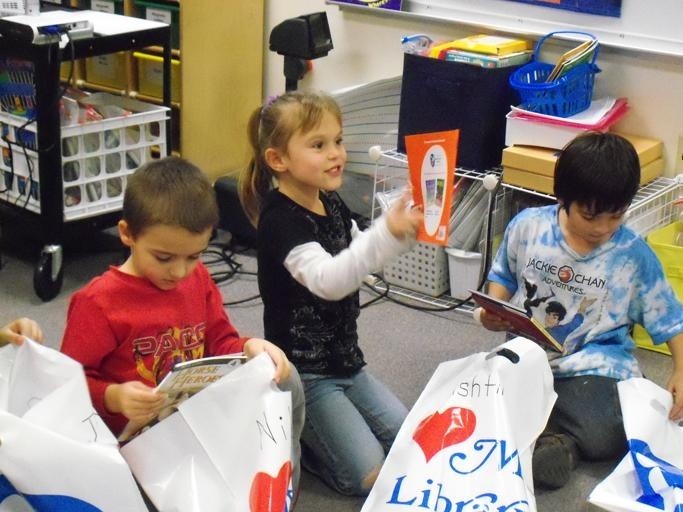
top-left (368, 145), bottom-right (683, 323)
top-left (40, 0), bottom-right (264, 186)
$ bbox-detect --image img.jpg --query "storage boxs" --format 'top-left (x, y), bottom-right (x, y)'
top-left (40, 0), bottom-right (182, 103)
top-left (633, 220), bottom-right (683, 356)
top-left (397, 54), bottom-right (535, 172)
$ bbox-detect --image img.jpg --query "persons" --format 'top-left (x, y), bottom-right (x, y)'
top-left (473, 131), bottom-right (682, 489)
top-left (542, 296), bottom-right (597, 347)
top-left (0, 317), bottom-right (45, 348)
top-left (238, 90), bottom-right (433, 495)
top-left (61, 157), bottom-right (306, 512)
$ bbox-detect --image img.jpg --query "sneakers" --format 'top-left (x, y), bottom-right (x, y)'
top-left (531, 433), bottom-right (577, 490)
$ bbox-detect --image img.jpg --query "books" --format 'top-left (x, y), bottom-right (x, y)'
top-left (400, 130), bottom-right (461, 252)
top-left (437, 29), bottom-right (634, 131)
top-left (459, 290), bottom-right (566, 355)
top-left (117, 351), bottom-right (247, 445)
top-left (57, 98), bottom-right (167, 209)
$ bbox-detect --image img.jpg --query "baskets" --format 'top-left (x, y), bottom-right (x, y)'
top-left (509, 30), bottom-right (599, 116)
